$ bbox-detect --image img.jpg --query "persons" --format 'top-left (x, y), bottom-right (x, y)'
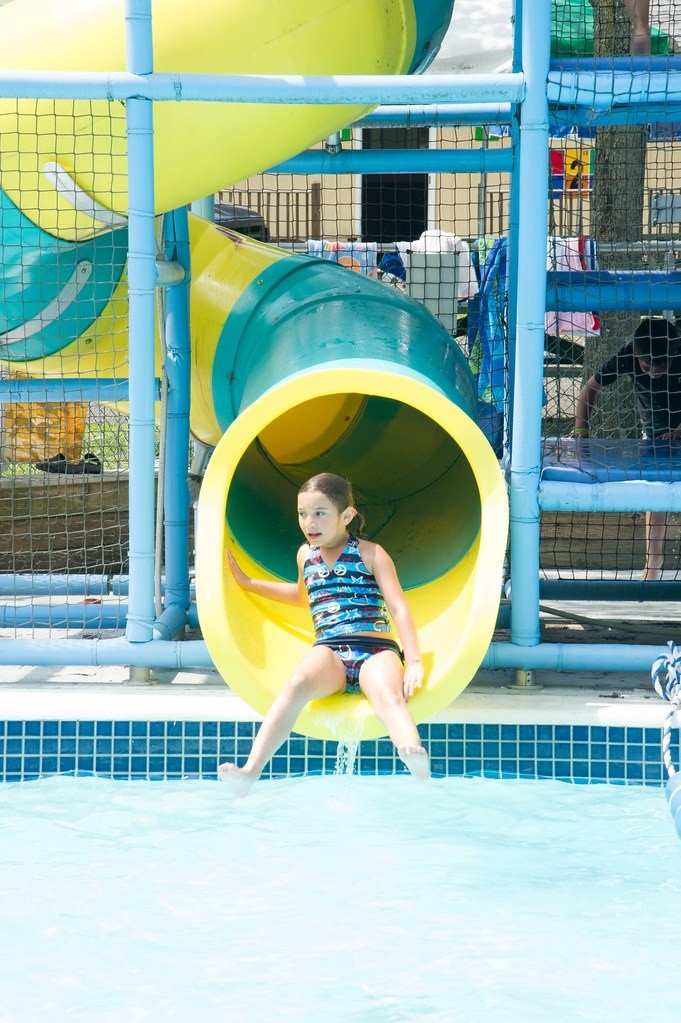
top-left (215, 472), bottom-right (432, 788)
top-left (625, 0), bottom-right (652, 55)
top-left (558, 316), bottom-right (681, 581)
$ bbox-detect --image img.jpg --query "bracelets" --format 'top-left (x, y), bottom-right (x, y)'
top-left (571, 427), bottom-right (589, 435)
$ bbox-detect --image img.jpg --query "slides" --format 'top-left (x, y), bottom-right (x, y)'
top-left (4, 0), bottom-right (673, 744)
